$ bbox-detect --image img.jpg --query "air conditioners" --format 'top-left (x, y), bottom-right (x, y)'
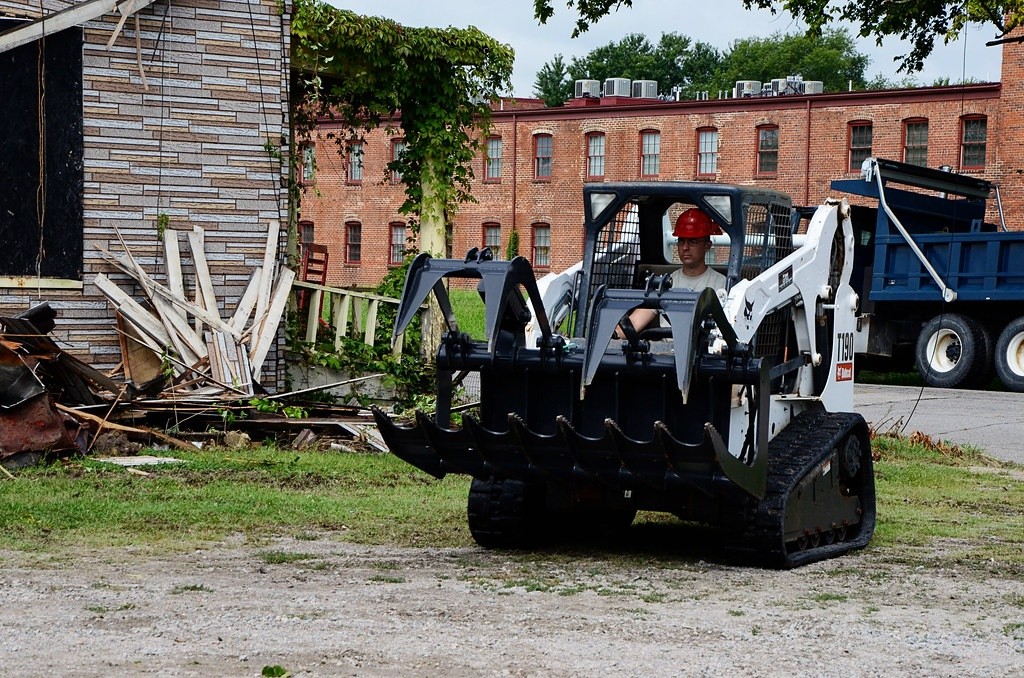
top-left (575, 76), bottom-right (824, 99)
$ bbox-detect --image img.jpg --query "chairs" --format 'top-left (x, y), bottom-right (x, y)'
top-left (298, 243), bottom-right (328, 329)
top-left (633, 264), bottom-right (760, 332)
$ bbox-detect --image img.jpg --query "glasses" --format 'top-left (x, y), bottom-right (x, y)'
top-left (677, 239), bottom-right (707, 245)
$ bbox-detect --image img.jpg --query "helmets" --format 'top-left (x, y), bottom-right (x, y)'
top-left (672, 208), bottom-right (712, 237)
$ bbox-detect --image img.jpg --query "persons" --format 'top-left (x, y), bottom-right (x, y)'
top-left (617, 209), bottom-right (728, 340)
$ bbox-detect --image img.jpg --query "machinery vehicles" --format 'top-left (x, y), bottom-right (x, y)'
top-left (364, 172), bottom-right (876, 565)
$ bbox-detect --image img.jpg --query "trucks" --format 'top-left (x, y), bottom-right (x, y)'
top-left (741, 161), bottom-right (1024, 389)
